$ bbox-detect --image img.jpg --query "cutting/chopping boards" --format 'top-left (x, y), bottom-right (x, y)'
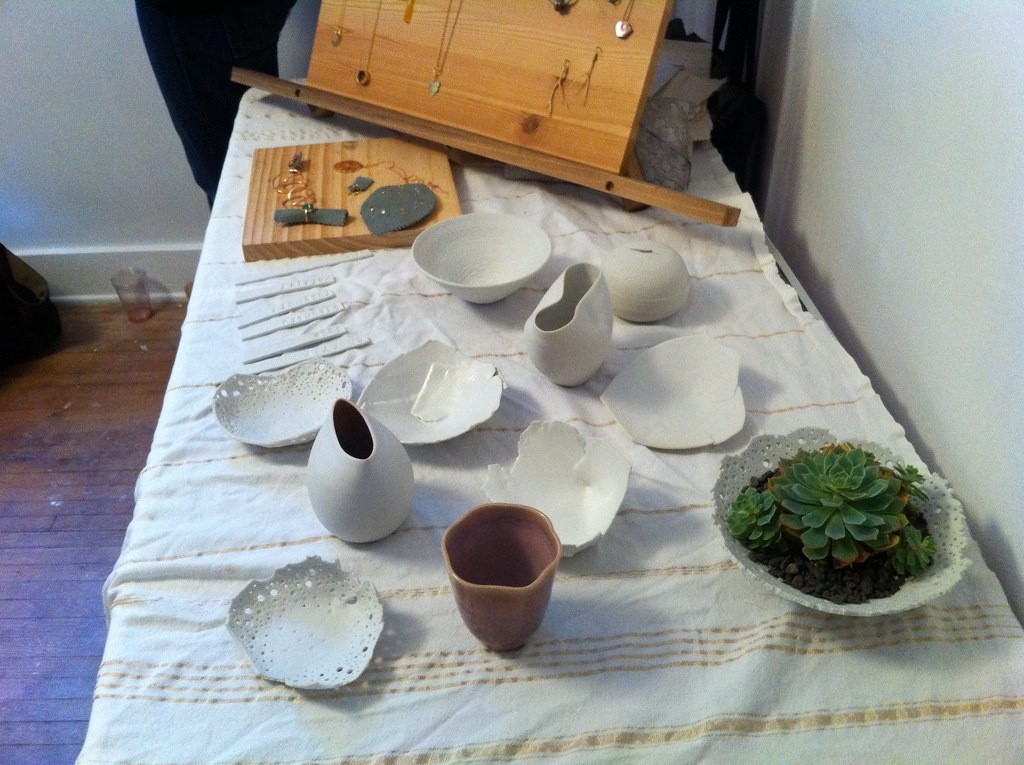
top-left (241, 135), bottom-right (463, 263)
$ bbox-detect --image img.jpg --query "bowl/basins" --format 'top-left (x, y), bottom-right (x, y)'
top-left (411, 213), bottom-right (552, 305)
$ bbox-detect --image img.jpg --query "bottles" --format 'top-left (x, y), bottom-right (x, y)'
top-left (306, 398), bottom-right (413, 544)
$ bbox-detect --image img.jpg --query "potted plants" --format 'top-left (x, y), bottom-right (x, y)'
top-left (712, 424), bottom-right (971, 615)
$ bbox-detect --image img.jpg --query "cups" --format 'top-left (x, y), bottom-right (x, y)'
top-left (111, 267), bottom-right (153, 322)
top-left (523, 262), bottom-right (613, 386)
top-left (441, 503), bottom-right (563, 653)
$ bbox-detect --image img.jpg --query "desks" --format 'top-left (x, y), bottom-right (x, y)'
top-left (78, 87), bottom-right (1023, 765)
